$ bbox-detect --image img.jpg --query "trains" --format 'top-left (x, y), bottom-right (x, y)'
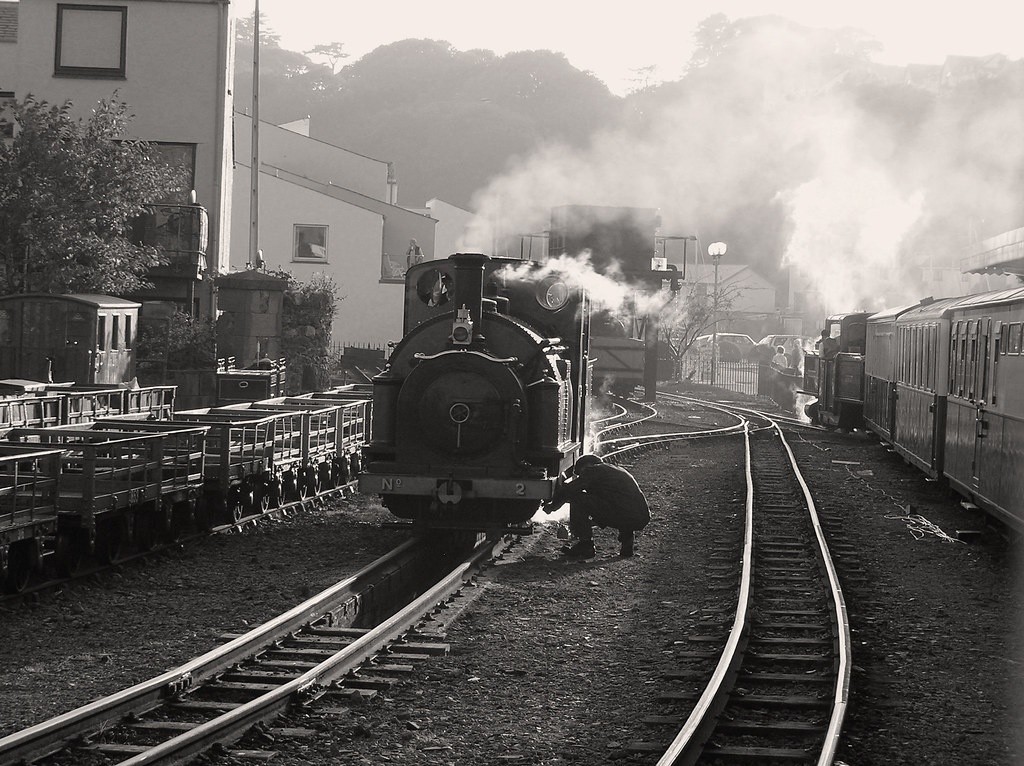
top-left (799, 289), bottom-right (1024, 536)
top-left (357, 251), bottom-right (599, 530)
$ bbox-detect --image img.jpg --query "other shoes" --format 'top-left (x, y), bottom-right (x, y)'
top-left (620, 543), bottom-right (634, 556)
top-left (572, 541), bottom-right (595, 558)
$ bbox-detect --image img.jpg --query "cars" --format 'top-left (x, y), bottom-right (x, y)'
top-left (688, 335), bottom-right (709, 354)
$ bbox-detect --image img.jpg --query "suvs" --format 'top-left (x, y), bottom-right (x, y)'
top-left (757, 334), bottom-right (815, 358)
top-left (707, 333), bottom-right (759, 361)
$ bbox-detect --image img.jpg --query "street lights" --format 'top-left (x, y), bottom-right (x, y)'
top-left (707, 242), bottom-right (728, 381)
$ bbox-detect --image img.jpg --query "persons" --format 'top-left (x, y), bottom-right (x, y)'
top-left (819, 330), bottom-right (840, 357)
top-left (772, 338), bottom-right (804, 369)
top-left (406, 238), bottom-right (424, 268)
top-left (543, 454), bottom-right (650, 557)
